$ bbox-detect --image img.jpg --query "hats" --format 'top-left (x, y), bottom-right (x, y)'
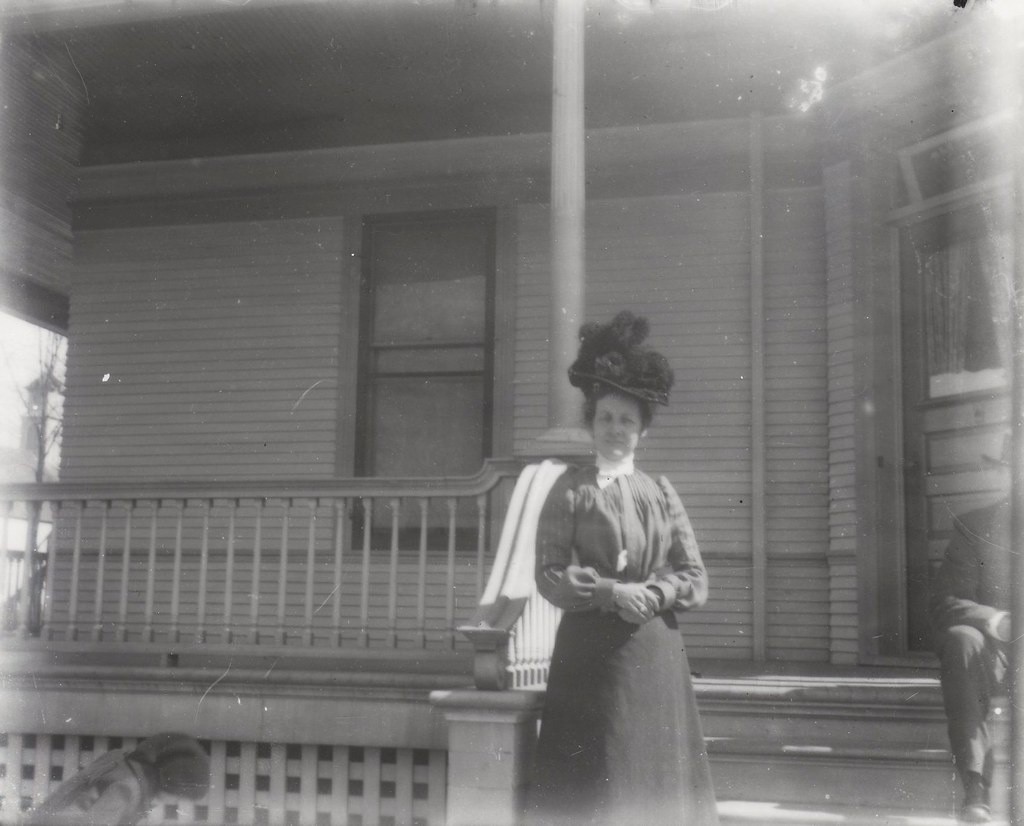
top-left (982, 434), bottom-right (1013, 466)
top-left (566, 311), bottom-right (674, 405)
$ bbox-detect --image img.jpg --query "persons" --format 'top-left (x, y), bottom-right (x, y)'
top-left (24, 731), bottom-right (210, 826)
top-left (932, 433), bottom-right (1013, 822)
top-left (529, 309), bottom-right (713, 826)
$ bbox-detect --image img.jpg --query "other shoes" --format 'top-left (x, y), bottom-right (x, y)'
top-left (960, 771), bottom-right (990, 822)
